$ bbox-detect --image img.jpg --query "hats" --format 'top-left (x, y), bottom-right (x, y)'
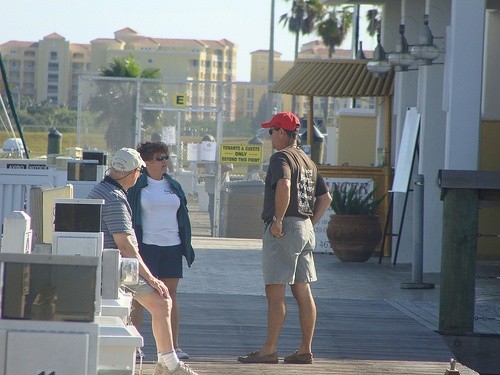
top-left (0, 138), bottom-right (30, 154)
top-left (261, 112), bottom-right (300, 131)
top-left (111, 147), bottom-right (146, 171)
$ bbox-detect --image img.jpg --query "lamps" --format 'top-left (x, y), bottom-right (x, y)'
top-left (409, 14), bottom-right (446, 66)
top-left (366, 33), bottom-right (394, 78)
top-left (387, 25), bottom-right (419, 71)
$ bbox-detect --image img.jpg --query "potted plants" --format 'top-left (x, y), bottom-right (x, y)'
top-left (327, 182), bottom-right (387, 263)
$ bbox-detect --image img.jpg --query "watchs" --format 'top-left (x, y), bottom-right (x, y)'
top-left (273, 216), bottom-right (283, 221)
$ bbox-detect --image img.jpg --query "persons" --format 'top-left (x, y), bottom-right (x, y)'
top-left (203, 135), bottom-right (233, 237)
top-left (127, 142), bottom-right (195, 359)
top-left (88, 147), bottom-right (198, 375)
top-left (238, 112), bottom-right (333, 363)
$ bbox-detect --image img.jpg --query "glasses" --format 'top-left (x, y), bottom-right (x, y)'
top-left (154, 155), bottom-right (168, 161)
top-left (269, 128), bottom-right (279, 135)
top-left (137, 167), bottom-right (144, 173)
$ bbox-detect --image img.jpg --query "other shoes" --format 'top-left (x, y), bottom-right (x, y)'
top-left (284, 350), bottom-right (314, 363)
top-left (176, 349), bottom-right (189, 359)
top-left (238, 350), bottom-right (279, 363)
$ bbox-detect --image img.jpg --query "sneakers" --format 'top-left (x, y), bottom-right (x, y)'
top-left (152, 361), bottom-right (199, 375)
